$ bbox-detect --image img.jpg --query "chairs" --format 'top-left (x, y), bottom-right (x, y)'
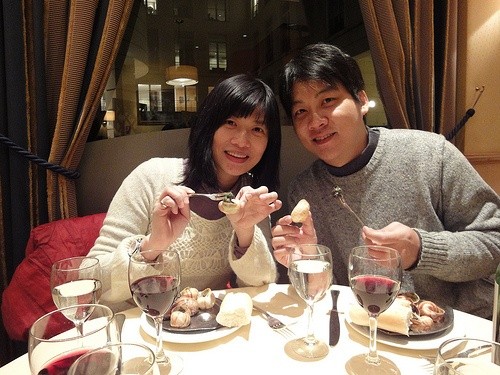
top-left (0, 211), bottom-right (106, 342)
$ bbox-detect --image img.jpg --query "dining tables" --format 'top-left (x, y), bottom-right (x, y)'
top-left (0, 284), bottom-right (493, 375)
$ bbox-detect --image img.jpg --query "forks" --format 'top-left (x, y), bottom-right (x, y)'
top-left (419, 344), bottom-right (492, 365)
top-left (188, 191), bottom-right (229, 201)
top-left (252, 305), bottom-right (295, 339)
top-left (337, 193), bottom-right (363, 228)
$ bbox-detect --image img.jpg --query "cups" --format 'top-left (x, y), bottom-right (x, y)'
top-left (434, 337), bottom-right (500, 375)
top-left (28, 304), bottom-right (117, 375)
top-left (68, 342), bottom-right (155, 375)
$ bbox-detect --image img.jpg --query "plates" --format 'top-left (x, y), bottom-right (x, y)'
top-left (140, 293), bottom-right (242, 345)
top-left (377, 292), bottom-right (455, 336)
top-left (344, 295), bottom-right (467, 350)
top-left (146, 298), bottom-right (223, 333)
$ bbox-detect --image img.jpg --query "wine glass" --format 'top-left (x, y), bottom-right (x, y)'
top-left (130, 250), bottom-right (183, 374)
top-left (344, 244), bottom-right (401, 375)
top-left (51, 256), bottom-right (103, 338)
top-left (284, 244), bottom-right (333, 362)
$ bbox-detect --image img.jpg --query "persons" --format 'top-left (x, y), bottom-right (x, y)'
top-left (79, 75), bottom-right (282, 317)
top-left (272, 44), bottom-right (500, 321)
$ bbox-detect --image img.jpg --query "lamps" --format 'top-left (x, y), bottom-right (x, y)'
top-left (167, 66), bottom-right (200, 87)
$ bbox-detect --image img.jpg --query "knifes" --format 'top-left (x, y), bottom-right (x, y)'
top-left (112, 314), bottom-right (126, 374)
top-left (328, 290), bottom-right (341, 347)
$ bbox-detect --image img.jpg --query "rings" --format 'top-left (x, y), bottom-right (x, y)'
top-left (160, 201), bottom-right (167, 209)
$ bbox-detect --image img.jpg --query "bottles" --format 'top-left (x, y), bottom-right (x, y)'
top-left (490, 263), bottom-right (500, 366)
